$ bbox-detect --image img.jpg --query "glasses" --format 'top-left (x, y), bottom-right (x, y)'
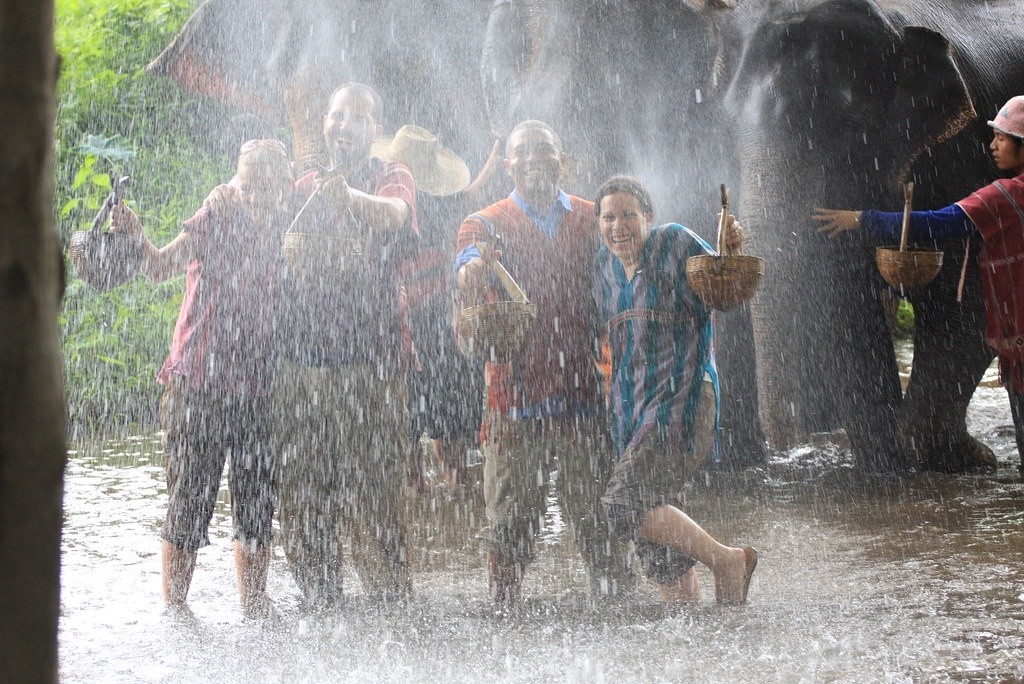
top-left (240, 139), bottom-right (287, 157)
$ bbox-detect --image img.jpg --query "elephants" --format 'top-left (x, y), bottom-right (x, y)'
top-left (138, 1), bottom-right (1024, 488)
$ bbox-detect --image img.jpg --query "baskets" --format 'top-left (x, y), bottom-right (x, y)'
top-left (284, 161), bottom-right (362, 278)
top-left (68, 169), bottom-right (144, 292)
top-left (875, 182), bottom-right (944, 291)
top-left (460, 234), bottom-right (537, 364)
top-left (686, 183), bottom-right (766, 312)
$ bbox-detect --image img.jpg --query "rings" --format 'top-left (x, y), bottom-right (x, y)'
top-left (734, 230), bottom-right (740, 236)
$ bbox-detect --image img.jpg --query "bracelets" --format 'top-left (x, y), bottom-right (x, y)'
top-left (853, 210), bottom-right (860, 227)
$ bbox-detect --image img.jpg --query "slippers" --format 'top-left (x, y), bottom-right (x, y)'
top-left (716, 545), bottom-right (757, 607)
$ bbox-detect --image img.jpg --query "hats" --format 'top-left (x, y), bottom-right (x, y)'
top-left (369, 125), bottom-right (471, 195)
top-left (987, 95), bottom-right (1024, 138)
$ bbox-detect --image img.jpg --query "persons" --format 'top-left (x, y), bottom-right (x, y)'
top-left (106, 82), bottom-right (748, 626)
top-left (809, 95), bottom-right (1024, 482)
